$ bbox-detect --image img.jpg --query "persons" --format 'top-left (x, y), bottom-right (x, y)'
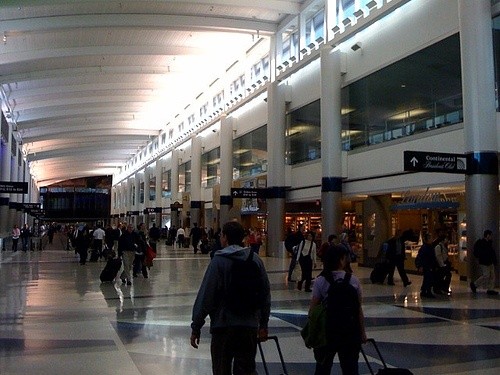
top-left (287, 222), bottom-right (314, 282)
top-left (444, 239), bottom-right (451, 258)
top-left (470, 230), bottom-right (500, 294)
top-left (295, 231), bottom-right (318, 292)
top-left (385, 230), bottom-right (412, 287)
top-left (337, 230), bottom-right (360, 275)
top-left (10, 219), bottom-right (264, 288)
top-left (431, 236), bottom-right (451, 297)
top-left (189, 220), bottom-right (271, 375)
top-left (415, 233), bottom-right (438, 299)
top-left (309, 244), bottom-right (368, 375)
top-left (432, 227), bottom-right (443, 245)
top-left (284, 221), bottom-right (296, 257)
top-left (320, 234), bottom-right (343, 279)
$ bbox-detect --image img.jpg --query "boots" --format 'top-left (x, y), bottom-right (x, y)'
top-left (133, 268), bottom-right (137, 277)
top-left (142, 265), bottom-right (148, 278)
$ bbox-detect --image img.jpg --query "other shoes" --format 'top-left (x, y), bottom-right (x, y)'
top-left (427, 292), bottom-right (434, 298)
top-left (470, 281), bottom-right (477, 294)
top-left (387, 280), bottom-right (395, 285)
top-left (420, 292), bottom-right (427, 297)
top-left (305, 288), bottom-right (311, 292)
top-left (120, 275), bottom-right (125, 283)
top-left (487, 290), bottom-right (496, 295)
top-left (298, 282), bottom-right (302, 290)
top-left (403, 281), bottom-right (412, 287)
top-left (127, 279), bottom-right (131, 285)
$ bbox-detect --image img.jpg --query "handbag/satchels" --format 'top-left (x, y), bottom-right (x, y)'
top-left (291, 262), bottom-right (302, 281)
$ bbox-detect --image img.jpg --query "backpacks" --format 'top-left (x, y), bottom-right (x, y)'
top-left (322, 270), bottom-right (361, 352)
top-left (216, 250), bottom-right (270, 310)
top-left (415, 246), bottom-right (424, 267)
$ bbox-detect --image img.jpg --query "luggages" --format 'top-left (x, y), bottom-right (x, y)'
top-left (361, 338), bottom-right (414, 375)
top-left (100, 258), bottom-right (122, 281)
top-left (371, 261), bottom-right (391, 284)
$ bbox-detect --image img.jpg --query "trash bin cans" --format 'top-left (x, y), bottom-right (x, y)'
top-left (278, 241), bottom-right (288, 258)
